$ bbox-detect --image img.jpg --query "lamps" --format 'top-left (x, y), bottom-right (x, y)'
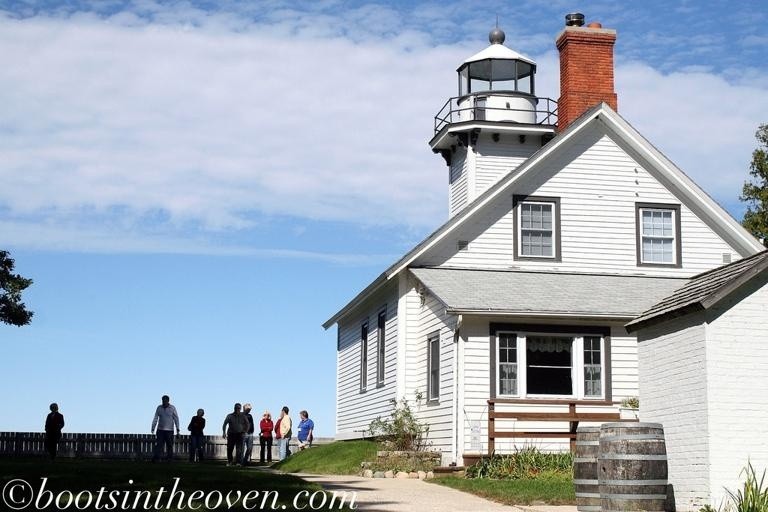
top-left (418, 287), bottom-right (428, 304)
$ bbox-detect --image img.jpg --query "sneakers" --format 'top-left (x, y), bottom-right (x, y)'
top-left (225, 461), bottom-right (272, 470)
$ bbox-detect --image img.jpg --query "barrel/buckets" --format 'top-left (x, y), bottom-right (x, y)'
top-left (596, 422), bottom-right (668, 512)
top-left (572, 427), bottom-right (602, 512)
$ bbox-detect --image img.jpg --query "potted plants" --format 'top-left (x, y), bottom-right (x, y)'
top-left (619, 396), bottom-right (639, 420)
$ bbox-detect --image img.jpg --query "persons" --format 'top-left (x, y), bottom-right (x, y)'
top-left (152, 395), bottom-right (179, 466)
top-left (222, 402), bottom-right (250, 465)
top-left (258, 408), bottom-right (274, 464)
top-left (296, 410), bottom-right (314, 450)
top-left (280, 406), bottom-right (292, 461)
top-left (187, 408), bottom-right (208, 464)
top-left (274, 410), bottom-right (291, 457)
top-left (45, 402), bottom-right (65, 464)
top-left (241, 404), bottom-right (254, 464)
